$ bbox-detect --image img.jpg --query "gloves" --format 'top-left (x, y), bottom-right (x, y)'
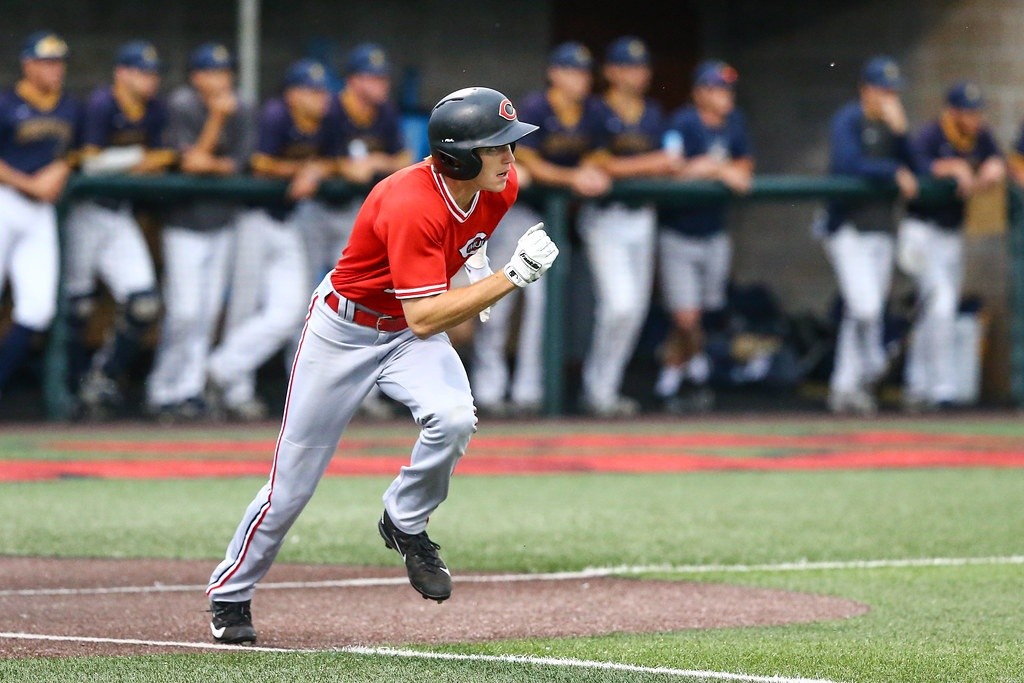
top-left (501, 222), bottom-right (559, 288)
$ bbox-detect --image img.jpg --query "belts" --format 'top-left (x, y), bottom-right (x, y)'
top-left (325, 292), bottom-right (409, 333)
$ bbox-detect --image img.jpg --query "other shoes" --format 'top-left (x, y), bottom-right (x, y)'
top-left (583, 394), bottom-right (639, 421)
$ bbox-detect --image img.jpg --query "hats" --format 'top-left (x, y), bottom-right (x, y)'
top-left (861, 56), bottom-right (903, 93)
top-left (607, 36), bottom-right (650, 66)
top-left (553, 42), bottom-right (595, 72)
top-left (192, 42), bottom-right (236, 70)
top-left (343, 43), bottom-right (398, 75)
top-left (115, 39), bottom-right (178, 75)
top-left (692, 61), bottom-right (738, 89)
top-left (283, 56), bottom-right (344, 94)
top-left (21, 32), bottom-right (82, 62)
top-left (941, 82), bottom-right (985, 110)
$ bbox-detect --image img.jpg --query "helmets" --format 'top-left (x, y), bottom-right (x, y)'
top-left (428, 88), bottom-right (540, 180)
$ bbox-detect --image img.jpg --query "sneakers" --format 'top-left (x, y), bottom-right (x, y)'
top-left (209, 599), bottom-right (258, 644)
top-left (654, 353), bottom-right (716, 415)
top-left (465, 240), bottom-right (498, 322)
top-left (378, 509), bottom-right (452, 604)
top-left (906, 387), bottom-right (955, 417)
top-left (207, 362), bottom-right (268, 426)
top-left (475, 400), bottom-right (545, 419)
top-left (147, 390), bottom-right (225, 421)
top-left (830, 385), bottom-right (878, 420)
top-left (36, 368), bottom-right (146, 422)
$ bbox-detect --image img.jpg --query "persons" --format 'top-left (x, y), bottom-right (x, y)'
top-left (472, 38), bottom-right (756, 416)
top-left (206, 87), bottom-right (559, 644)
top-left (818, 55), bottom-right (1024, 414)
top-left (0, 36), bottom-right (410, 419)
